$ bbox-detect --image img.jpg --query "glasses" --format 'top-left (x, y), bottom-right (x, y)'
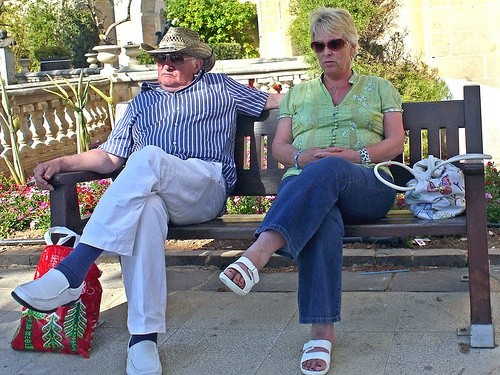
top-left (310, 39), bottom-right (349, 52)
top-left (154, 53), bottom-right (195, 67)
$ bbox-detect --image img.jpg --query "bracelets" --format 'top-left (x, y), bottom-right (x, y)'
top-left (294, 150), bottom-right (302, 169)
top-left (358, 148), bottom-right (371, 163)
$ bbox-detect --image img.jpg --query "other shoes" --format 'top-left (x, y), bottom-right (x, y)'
top-left (11, 267), bottom-right (86, 313)
top-left (126, 336), bottom-right (162, 375)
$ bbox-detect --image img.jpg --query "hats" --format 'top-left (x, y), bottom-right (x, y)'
top-left (140, 26), bottom-right (215, 72)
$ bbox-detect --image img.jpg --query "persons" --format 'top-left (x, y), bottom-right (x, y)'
top-left (11, 27), bottom-right (285, 375)
top-left (219, 9), bottom-right (404, 375)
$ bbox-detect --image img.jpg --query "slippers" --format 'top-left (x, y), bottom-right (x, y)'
top-left (299, 339), bottom-right (332, 375)
top-left (219, 256), bottom-right (260, 295)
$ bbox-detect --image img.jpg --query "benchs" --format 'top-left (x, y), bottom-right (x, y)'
top-left (49, 85), bottom-right (495, 348)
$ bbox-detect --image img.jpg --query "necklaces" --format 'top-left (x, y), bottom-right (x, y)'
top-left (332, 86), bottom-right (336, 94)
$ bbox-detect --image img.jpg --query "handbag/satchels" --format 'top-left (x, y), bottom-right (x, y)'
top-left (10, 226), bottom-right (103, 359)
top-left (373, 153), bottom-right (492, 220)
